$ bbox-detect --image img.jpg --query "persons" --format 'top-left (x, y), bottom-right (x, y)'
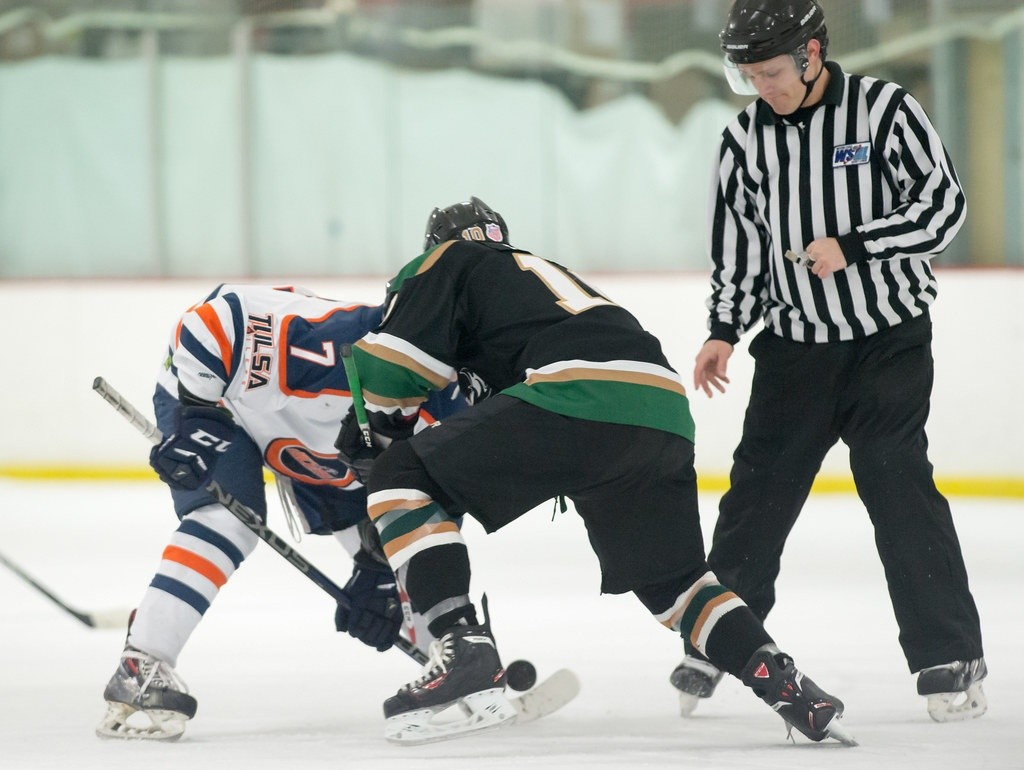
top-left (671, 2), bottom-right (988, 699)
top-left (340, 197), bottom-right (844, 743)
top-left (101, 283), bottom-right (471, 716)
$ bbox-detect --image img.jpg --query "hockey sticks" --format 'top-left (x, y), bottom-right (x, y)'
top-left (0, 551), bottom-right (139, 634)
top-left (94, 377), bottom-right (580, 728)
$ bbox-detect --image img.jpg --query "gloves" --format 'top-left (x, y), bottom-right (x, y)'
top-left (335, 550), bottom-right (404, 650)
top-left (150, 403), bottom-right (236, 491)
top-left (335, 407), bottom-right (382, 482)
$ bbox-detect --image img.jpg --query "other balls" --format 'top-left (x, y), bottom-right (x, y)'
top-left (505, 661), bottom-right (537, 691)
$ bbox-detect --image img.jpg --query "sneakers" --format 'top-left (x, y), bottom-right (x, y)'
top-left (670, 653), bottom-right (726, 716)
top-left (916, 655), bottom-right (990, 725)
top-left (96, 641), bottom-right (197, 741)
top-left (381, 627), bottom-right (518, 743)
top-left (740, 644), bottom-right (859, 747)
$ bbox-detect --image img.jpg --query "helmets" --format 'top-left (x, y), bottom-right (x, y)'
top-left (720, 0), bottom-right (830, 70)
top-left (423, 197), bottom-right (509, 255)
